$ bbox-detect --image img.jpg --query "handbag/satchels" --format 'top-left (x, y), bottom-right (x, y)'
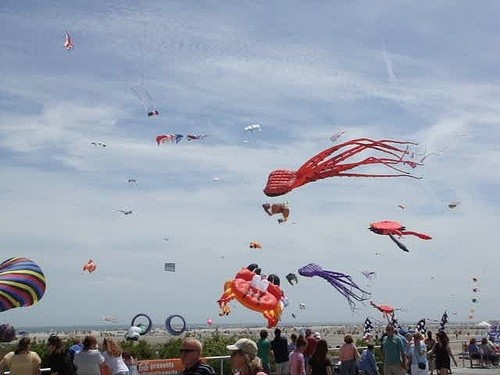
top-left (419, 362), bottom-right (426, 370)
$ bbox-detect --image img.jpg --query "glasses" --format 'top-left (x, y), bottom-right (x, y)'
top-left (179, 349), bottom-right (198, 353)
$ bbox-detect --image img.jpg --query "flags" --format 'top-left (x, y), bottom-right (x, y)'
top-left (364, 316), bottom-right (381, 333)
top-left (438, 310), bottom-right (448, 331)
top-left (414, 318), bottom-right (425, 332)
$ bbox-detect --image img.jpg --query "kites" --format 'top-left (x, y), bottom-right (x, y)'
top-left (285, 272), bottom-right (298, 286)
top-left (187, 133), bottom-right (207, 142)
top-left (155, 133), bottom-right (185, 146)
top-left (249, 240), bottom-right (263, 250)
top-left (217, 262), bottom-right (290, 328)
top-left (369, 300), bottom-right (407, 325)
top-left (262, 202), bottom-right (291, 225)
top-left (243, 123), bottom-right (262, 131)
top-left (299, 302), bottom-right (306, 311)
top-left (62, 30), bottom-right (75, 52)
top-left (447, 200), bottom-right (465, 209)
top-left (113, 208), bottom-right (136, 218)
top-left (261, 136), bottom-right (428, 197)
top-left (163, 263), bottom-right (177, 273)
top-left (89, 140), bottom-right (107, 148)
top-left (0, 257), bottom-right (46, 315)
top-left (469, 278), bottom-right (479, 321)
top-left (81, 258), bottom-right (97, 274)
top-left (397, 203), bottom-right (407, 211)
top-left (147, 110), bottom-right (160, 117)
top-left (330, 129), bottom-right (344, 142)
top-left (366, 220), bottom-right (432, 253)
top-left (297, 263), bottom-right (373, 314)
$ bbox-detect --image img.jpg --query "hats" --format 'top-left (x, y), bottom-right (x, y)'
top-left (314, 332), bottom-right (321, 339)
top-left (225, 338), bottom-right (258, 355)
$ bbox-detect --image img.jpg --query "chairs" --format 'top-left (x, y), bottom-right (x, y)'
top-left (460, 341), bottom-right (484, 368)
top-left (478, 344), bottom-right (500, 368)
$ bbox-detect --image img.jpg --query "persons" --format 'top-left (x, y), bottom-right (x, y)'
top-left (255, 329), bottom-right (272, 375)
top-left (303, 329), bottom-right (318, 375)
top-left (287, 334), bottom-right (297, 352)
top-left (101, 337), bottom-right (130, 375)
top-left (123, 323), bottom-right (142, 340)
top-left (488, 324), bottom-right (500, 343)
top-left (424, 331), bottom-right (436, 375)
top-left (469, 337), bottom-right (485, 368)
top-left (178, 337), bottom-right (216, 375)
top-left (289, 335), bottom-right (306, 375)
top-left (41, 333), bottom-right (77, 375)
top-left (313, 331), bottom-right (322, 343)
top-left (225, 338), bottom-right (267, 375)
top-left (73, 334), bottom-right (105, 375)
top-left (480, 337), bottom-right (496, 368)
top-left (270, 328), bottom-right (289, 375)
top-left (381, 324), bottom-right (407, 375)
top-left (122, 352), bottom-right (139, 375)
top-left (394, 330), bottom-right (408, 355)
top-left (401, 332), bottom-right (429, 375)
top-left (404, 334), bottom-right (412, 375)
top-left (427, 331), bottom-right (458, 375)
top-left (68, 337), bottom-right (84, 354)
top-left (358, 343), bottom-right (380, 375)
top-left (306, 339), bottom-right (333, 375)
top-left (0, 336), bottom-right (41, 375)
top-left (363, 333), bottom-right (372, 342)
top-left (338, 335), bottom-right (361, 375)
